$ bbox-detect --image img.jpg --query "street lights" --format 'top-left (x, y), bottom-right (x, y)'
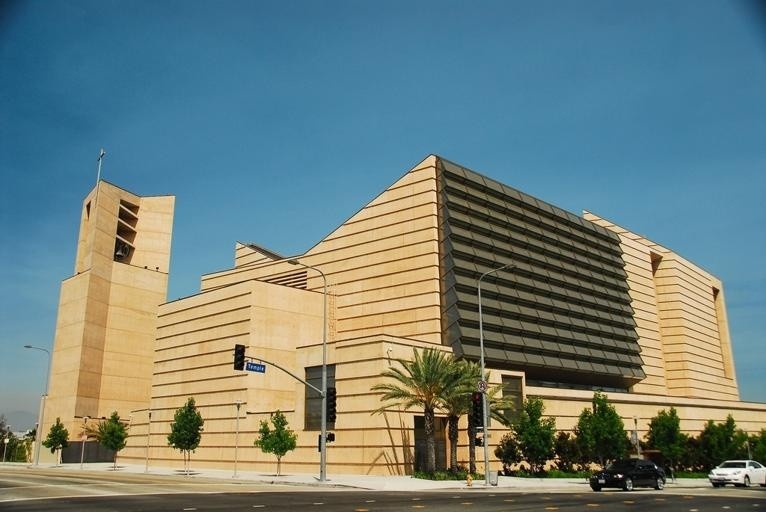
top-left (739, 424), bottom-right (753, 458)
top-left (630, 412), bottom-right (642, 459)
top-left (0, 416), bottom-right (90, 469)
top-left (477, 261), bottom-right (518, 487)
top-left (287, 257), bottom-right (329, 483)
top-left (21, 344), bottom-right (52, 465)
top-left (228, 399), bottom-right (246, 479)
top-left (143, 410), bottom-right (156, 474)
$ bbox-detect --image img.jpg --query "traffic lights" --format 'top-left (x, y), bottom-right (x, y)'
top-left (472, 390), bottom-right (483, 428)
top-left (326, 386), bottom-right (337, 423)
top-left (233, 343), bottom-right (246, 372)
top-left (328, 433), bottom-right (335, 442)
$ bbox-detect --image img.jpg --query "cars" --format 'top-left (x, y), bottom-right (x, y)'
top-left (707, 459), bottom-right (766, 489)
top-left (586, 458), bottom-right (668, 493)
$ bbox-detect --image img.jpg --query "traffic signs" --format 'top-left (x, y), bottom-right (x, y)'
top-left (244, 361), bottom-right (266, 373)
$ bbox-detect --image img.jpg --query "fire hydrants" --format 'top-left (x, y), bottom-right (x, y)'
top-left (466, 473), bottom-right (473, 487)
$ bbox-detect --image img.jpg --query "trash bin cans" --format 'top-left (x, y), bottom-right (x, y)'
top-left (489, 471), bottom-right (498, 487)
